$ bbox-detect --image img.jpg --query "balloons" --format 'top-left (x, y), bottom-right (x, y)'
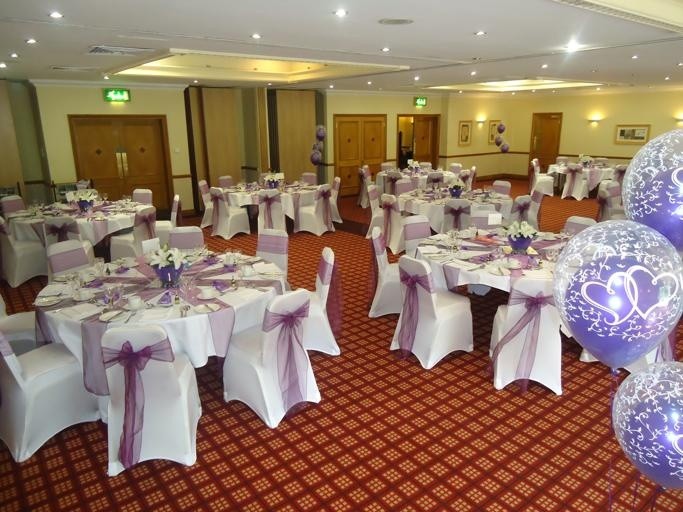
top-left (317, 126), bottom-right (325, 141)
top-left (495, 136), bottom-right (503, 147)
top-left (312, 142), bottom-right (323, 153)
top-left (621, 129), bottom-right (683, 255)
top-left (611, 361), bottom-right (683, 490)
top-left (496, 123), bottom-right (506, 135)
top-left (309, 150), bottom-right (322, 166)
top-left (502, 143), bottom-right (509, 153)
top-left (551, 219), bottom-right (683, 374)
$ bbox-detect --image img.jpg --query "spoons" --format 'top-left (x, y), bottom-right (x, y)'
top-left (124, 311), bottom-right (136, 323)
top-left (178, 304), bottom-right (189, 318)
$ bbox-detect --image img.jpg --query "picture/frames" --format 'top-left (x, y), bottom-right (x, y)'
top-left (614, 123), bottom-right (651, 146)
top-left (458, 120), bottom-right (473, 147)
top-left (487, 119), bottom-right (502, 145)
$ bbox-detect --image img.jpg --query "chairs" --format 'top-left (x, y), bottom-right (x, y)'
top-left (0, 334), bottom-right (100, 463)
top-left (222, 288), bottom-right (321, 429)
top-left (579, 326), bottom-right (677, 375)
top-left (367, 227), bottom-right (404, 318)
top-left (488, 276), bottom-right (566, 396)
top-left (47, 239), bottom-right (90, 277)
top-left (256, 229), bottom-right (289, 281)
top-left (285, 246), bottom-right (341, 356)
top-left (390, 256), bottom-right (474, 370)
top-left (1, 218), bottom-right (47, 289)
top-left (1, 156), bottom-right (628, 255)
top-left (99, 324), bottom-right (202, 475)
top-left (0, 298), bottom-right (35, 356)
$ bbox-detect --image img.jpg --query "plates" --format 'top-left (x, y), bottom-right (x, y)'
top-left (99, 309), bottom-right (127, 322)
top-left (422, 225), bottom-right (574, 276)
top-left (74, 293), bottom-right (96, 301)
top-left (34, 295), bottom-right (62, 306)
top-left (122, 301), bottom-right (146, 311)
top-left (193, 303), bottom-right (221, 314)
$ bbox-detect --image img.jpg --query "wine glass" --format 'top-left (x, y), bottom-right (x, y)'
top-left (94, 256), bottom-right (106, 280)
top-left (102, 279), bottom-right (126, 309)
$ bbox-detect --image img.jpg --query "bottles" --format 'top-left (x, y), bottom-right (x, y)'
top-left (174, 294), bottom-right (179, 304)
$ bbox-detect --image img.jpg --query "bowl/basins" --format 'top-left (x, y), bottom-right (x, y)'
top-left (14, 192), bottom-right (141, 223)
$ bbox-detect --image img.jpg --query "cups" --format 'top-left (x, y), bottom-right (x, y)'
top-left (179, 242), bottom-right (260, 305)
top-left (127, 296), bottom-right (141, 306)
top-left (77, 288), bottom-right (90, 299)
